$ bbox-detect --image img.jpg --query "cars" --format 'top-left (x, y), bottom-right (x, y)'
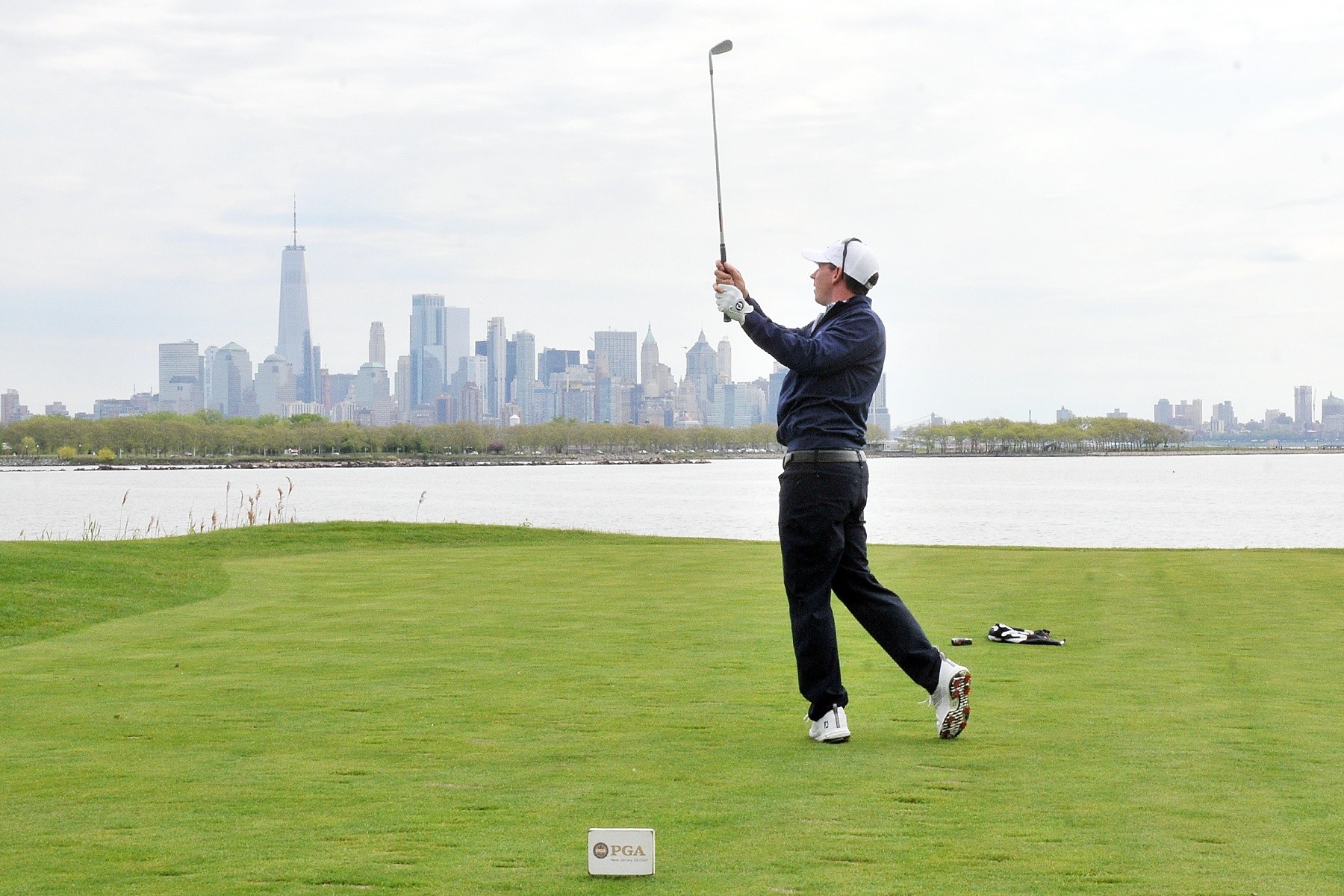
top-left (638, 450), bottom-right (648, 455)
top-left (660, 449), bottom-right (775, 454)
top-left (593, 450), bottom-right (603, 455)
top-left (532, 451), bottom-right (541, 455)
top-left (514, 451), bottom-right (524, 455)
top-left (170, 452), bottom-right (233, 458)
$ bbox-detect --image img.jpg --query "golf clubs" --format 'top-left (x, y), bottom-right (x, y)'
top-left (708, 39), bottom-right (733, 323)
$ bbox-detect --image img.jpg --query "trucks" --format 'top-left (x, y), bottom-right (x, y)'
top-left (284, 448), bottom-right (298, 455)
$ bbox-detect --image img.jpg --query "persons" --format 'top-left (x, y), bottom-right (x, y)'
top-left (713, 237), bottom-right (971, 742)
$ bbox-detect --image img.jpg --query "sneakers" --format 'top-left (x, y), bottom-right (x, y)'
top-left (931, 659), bottom-right (971, 739)
top-left (809, 706), bottom-right (851, 744)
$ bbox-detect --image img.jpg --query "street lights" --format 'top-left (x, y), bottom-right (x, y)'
top-left (79, 444), bottom-right (82, 455)
top-left (36, 445), bottom-right (39, 455)
top-left (26, 445), bottom-right (28, 455)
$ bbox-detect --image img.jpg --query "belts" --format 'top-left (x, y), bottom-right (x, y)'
top-left (783, 449), bottom-right (867, 468)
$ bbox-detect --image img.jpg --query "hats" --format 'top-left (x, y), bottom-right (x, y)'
top-left (802, 237), bottom-right (878, 290)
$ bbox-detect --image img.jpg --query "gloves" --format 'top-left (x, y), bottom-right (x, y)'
top-left (716, 285), bottom-right (754, 326)
